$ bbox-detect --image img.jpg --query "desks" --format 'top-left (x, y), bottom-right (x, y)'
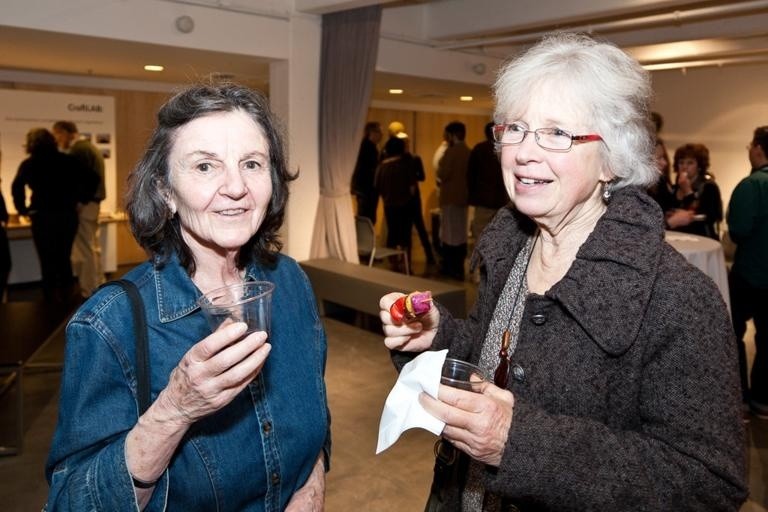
top-left (0, 210), bottom-right (131, 286)
top-left (664, 228), bottom-right (732, 332)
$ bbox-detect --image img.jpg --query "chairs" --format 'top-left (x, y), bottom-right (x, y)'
top-left (354, 216), bottom-right (411, 277)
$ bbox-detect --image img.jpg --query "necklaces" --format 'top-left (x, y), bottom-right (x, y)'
top-left (494, 336), bottom-right (512, 389)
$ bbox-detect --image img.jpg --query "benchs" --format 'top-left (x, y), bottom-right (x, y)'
top-left (300, 256), bottom-right (471, 338)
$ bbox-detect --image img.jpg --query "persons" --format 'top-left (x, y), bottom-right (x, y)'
top-left (650, 108), bottom-right (669, 157)
top-left (726, 124), bottom-right (768, 420)
top-left (354, 114), bottom-right (509, 283)
top-left (53, 120), bottom-right (105, 309)
top-left (645, 137), bottom-right (695, 234)
top-left (0, 155), bottom-right (12, 302)
top-left (44, 69), bottom-right (337, 512)
top-left (13, 128), bottom-right (95, 322)
top-left (383, 39), bottom-right (748, 509)
top-left (673, 144), bottom-right (723, 245)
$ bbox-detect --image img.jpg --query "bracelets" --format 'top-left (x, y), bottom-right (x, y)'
top-left (125, 463), bottom-right (169, 489)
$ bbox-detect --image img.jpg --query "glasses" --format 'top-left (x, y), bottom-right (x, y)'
top-left (492, 124), bottom-right (601, 151)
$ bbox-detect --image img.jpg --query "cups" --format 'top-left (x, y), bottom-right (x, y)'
top-left (193, 279), bottom-right (275, 351)
top-left (440, 357), bottom-right (487, 396)
